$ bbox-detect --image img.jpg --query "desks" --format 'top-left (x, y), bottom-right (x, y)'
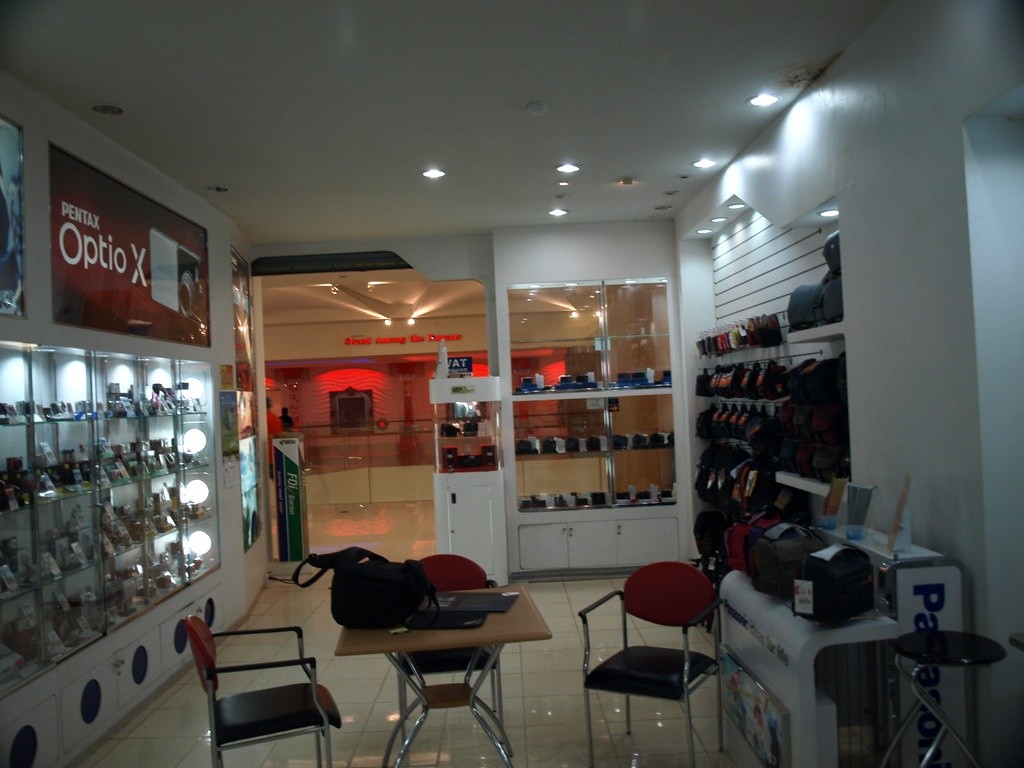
top-left (332, 586), bottom-right (552, 767)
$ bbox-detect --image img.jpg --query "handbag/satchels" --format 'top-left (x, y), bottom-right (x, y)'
top-left (294, 545), bottom-right (441, 631)
top-left (724, 511), bottom-right (875, 627)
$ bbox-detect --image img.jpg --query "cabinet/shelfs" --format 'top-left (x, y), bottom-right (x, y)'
top-left (0, 357), bottom-right (222, 768)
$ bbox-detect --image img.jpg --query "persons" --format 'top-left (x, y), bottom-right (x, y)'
top-left (278, 406), bottom-right (294, 432)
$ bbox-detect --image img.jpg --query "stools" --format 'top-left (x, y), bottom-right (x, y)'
top-left (873, 628), bottom-right (1008, 767)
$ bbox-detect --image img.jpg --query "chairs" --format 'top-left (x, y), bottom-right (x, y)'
top-left (397, 548), bottom-right (507, 733)
top-left (577, 558), bottom-right (725, 767)
top-left (181, 609), bottom-right (342, 768)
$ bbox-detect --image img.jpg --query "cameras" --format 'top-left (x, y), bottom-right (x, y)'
top-left (7, 456), bottom-right (23, 472)
top-left (149, 227), bottom-right (201, 326)
